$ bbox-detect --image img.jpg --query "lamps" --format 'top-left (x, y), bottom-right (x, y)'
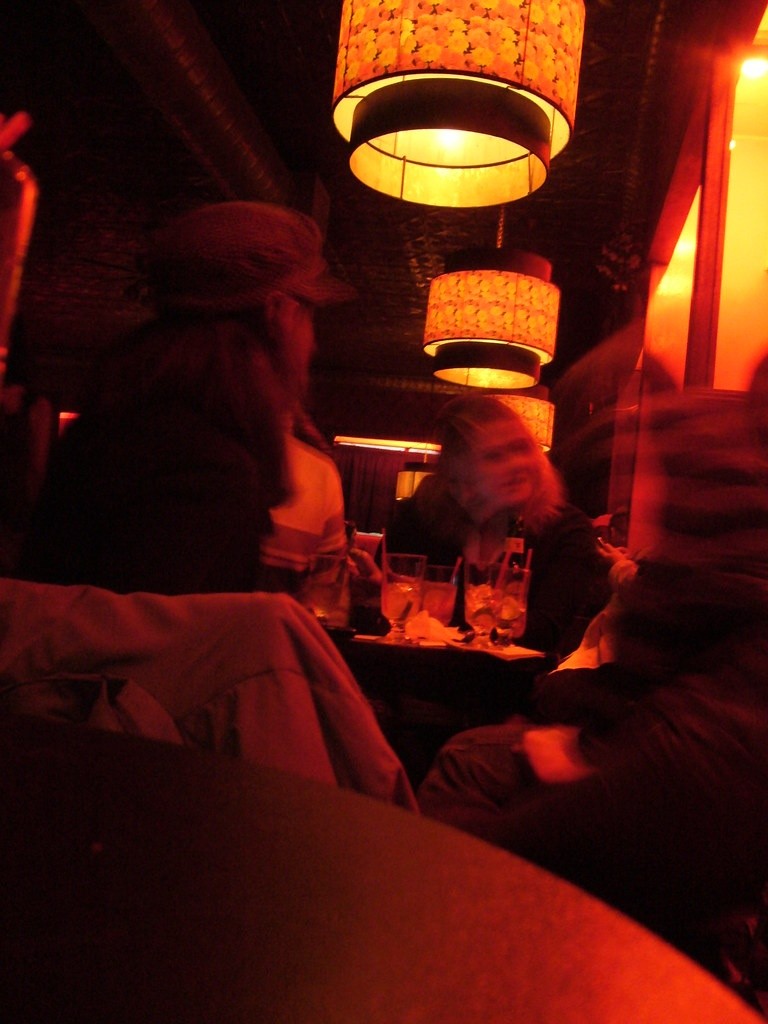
top-left (334, 0), bottom-right (586, 501)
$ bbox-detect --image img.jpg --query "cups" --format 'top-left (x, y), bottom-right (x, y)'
top-left (416, 563), bottom-right (457, 625)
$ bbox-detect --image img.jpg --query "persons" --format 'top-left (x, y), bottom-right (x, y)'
top-left (417, 343), bottom-right (768, 932)
top-left (347, 391), bottom-right (612, 786)
top-left (15, 199), bottom-right (352, 665)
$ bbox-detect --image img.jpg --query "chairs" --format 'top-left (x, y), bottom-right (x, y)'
top-left (0, 583), bottom-right (315, 788)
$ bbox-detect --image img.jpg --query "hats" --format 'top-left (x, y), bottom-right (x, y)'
top-left (146, 201), bottom-right (359, 311)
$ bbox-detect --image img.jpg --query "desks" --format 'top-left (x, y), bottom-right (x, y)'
top-left (316, 622), bottom-right (546, 795)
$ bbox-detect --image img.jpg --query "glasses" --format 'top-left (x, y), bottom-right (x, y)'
top-left (585, 511), bottom-right (631, 562)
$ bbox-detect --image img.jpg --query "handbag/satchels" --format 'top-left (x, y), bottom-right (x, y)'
top-left (0, 674), bottom-right (186, 745)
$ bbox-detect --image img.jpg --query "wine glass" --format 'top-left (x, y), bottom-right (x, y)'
top-left (374, 553), bottom-right (428, 647)
top-left (456, 546), bottom-right (533, 656)
